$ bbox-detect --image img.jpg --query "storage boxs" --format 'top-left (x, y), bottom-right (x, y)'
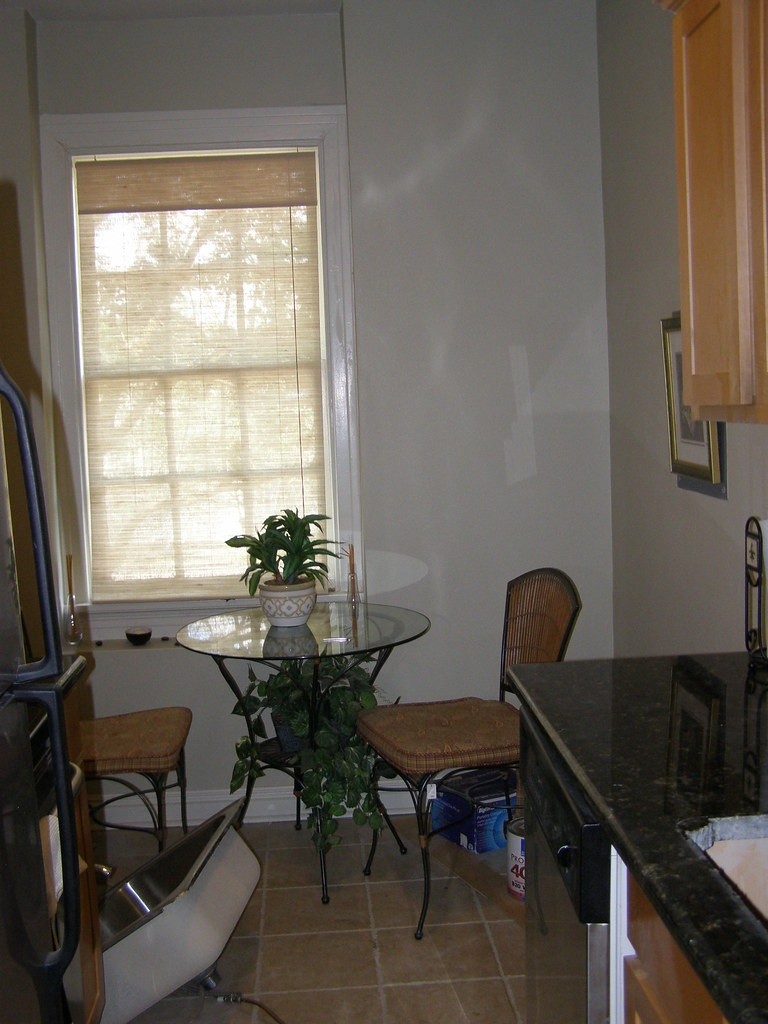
top-left (430, 769), bottom-right (516, 854)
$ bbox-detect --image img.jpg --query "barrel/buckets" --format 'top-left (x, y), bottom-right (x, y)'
top-left (507, 818), bottom-right (527, 899)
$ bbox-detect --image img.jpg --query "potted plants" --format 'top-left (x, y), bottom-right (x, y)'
top-left (225, 507), bottom-right (346, 627)
top-left (229, 643), bottom-right (402, 854)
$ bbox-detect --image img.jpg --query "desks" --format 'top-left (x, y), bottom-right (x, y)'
top-left (176, 601), bottom-right (431, 904)
top-left (506, 652), bottom-right (768, 1024)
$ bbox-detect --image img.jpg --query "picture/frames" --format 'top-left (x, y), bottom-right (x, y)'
top-left (659, 318), bottom-right (720, 485)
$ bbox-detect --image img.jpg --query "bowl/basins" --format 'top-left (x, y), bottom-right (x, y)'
top-left (126, 628), bottom-right (152, 646)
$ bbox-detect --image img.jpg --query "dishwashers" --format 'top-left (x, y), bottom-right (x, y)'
top-left (519, 706), bottom-right (606, 1024)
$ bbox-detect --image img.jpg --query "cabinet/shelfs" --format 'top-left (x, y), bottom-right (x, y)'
top-left (671, 0), bottom-right (767, 424)
top-left (517, 706), bottom-right (627, 1024)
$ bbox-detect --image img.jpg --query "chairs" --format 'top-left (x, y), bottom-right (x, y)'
top-left (79, 707), bottom-right (193, 856)
top-left (356, 568), bottom-right (581, 939)
